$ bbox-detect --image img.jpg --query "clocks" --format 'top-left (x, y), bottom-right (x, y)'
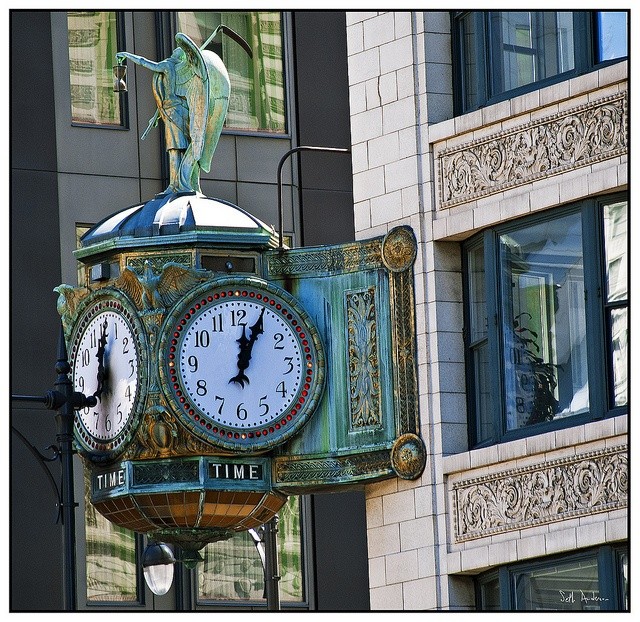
top-left (68, 286), bottom-right (151, 467)
top-left (155, 274), bottom-right (327, 454)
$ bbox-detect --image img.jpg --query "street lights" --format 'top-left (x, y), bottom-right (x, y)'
top-left (141, 526), bottom-right (281, 610)
